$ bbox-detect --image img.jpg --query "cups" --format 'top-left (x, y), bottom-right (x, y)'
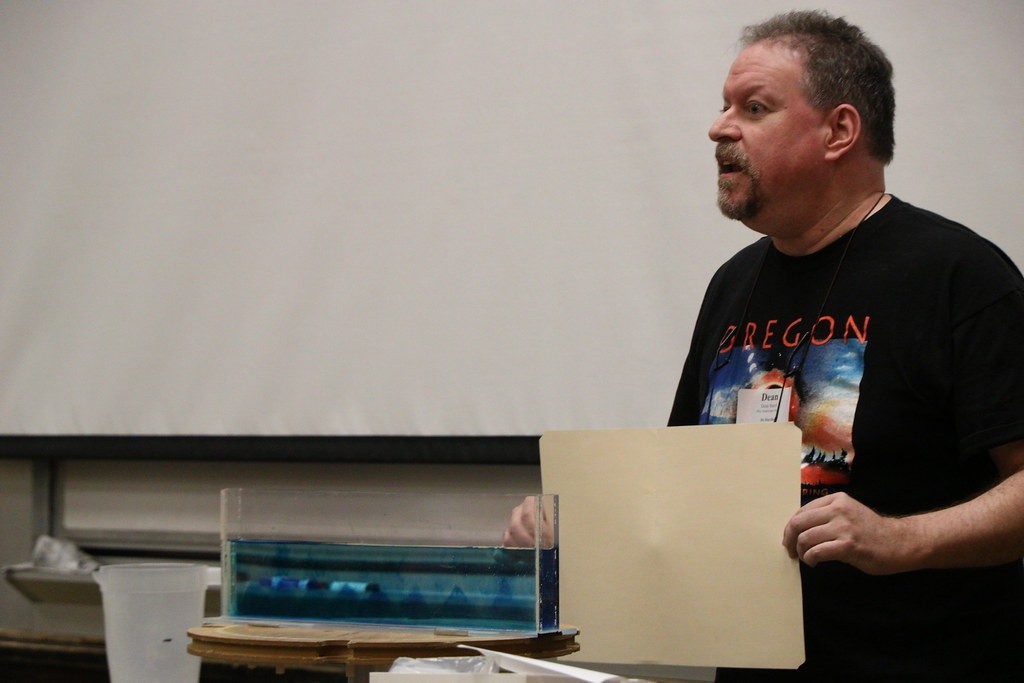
top-left (92, 562), bottom-right (209, 683)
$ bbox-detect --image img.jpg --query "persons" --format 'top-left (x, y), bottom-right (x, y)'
top-left (504, 8), bottom-right (1024, 683)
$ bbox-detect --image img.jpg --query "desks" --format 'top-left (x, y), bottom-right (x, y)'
top-left (186, 622), bottom-right (582, 683)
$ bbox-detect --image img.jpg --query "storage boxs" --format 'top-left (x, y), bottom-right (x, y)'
top-left (220, 487), bottom-right (560, 638)
